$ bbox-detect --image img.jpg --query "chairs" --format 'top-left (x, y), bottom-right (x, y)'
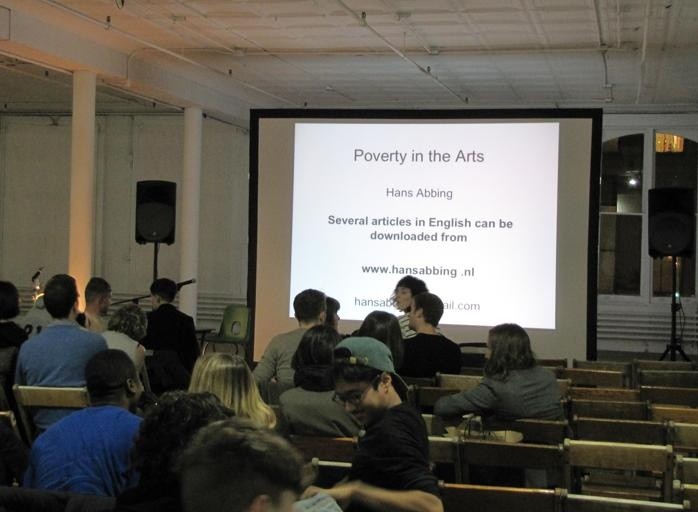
top-left (12, 384), bottom-right (95, 450)
top-left (263, 356), bottom-right (698, 511)
top-left (0, 410), bottom-right (23, 446)
top-left (195, 304), bottom-right (250, 359)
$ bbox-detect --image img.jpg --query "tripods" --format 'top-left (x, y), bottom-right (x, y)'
top-left (658, 256), bottom-right (690, 362)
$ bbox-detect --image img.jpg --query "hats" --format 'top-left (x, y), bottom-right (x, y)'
top-left (331, 336), bottom-right (410, 397)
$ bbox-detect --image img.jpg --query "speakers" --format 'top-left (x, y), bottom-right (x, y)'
top-left (135, 181), bottom-right (177, 246)
top-left (648, 187), bottom-right (695, 260)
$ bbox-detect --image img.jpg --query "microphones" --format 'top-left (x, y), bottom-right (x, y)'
top-left (177, 278), bottom-right (196, 287)
top-left (32, 267), bottom-right (45, 280)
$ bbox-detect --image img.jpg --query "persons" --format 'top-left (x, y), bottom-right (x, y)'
top-left (177, 420), bottom-right (343, 512)
top-left (114, 389), bottom-right (237, 511)
top-left (3, 269), bottom-right (563, 462)
top-left (301, 338), bottom-right (443, 512)
top-left (18, 350), bottom-right (146, 497)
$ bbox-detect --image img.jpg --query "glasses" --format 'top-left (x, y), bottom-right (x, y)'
top-left (331, 375), bottom-right (381, 410)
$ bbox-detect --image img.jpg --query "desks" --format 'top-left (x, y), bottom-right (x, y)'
top-left (194, 325), bottom-right (215, 351)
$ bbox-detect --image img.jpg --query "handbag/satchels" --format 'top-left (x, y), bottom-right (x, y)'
top-left (437, 424), bottom-right (526, 486)
